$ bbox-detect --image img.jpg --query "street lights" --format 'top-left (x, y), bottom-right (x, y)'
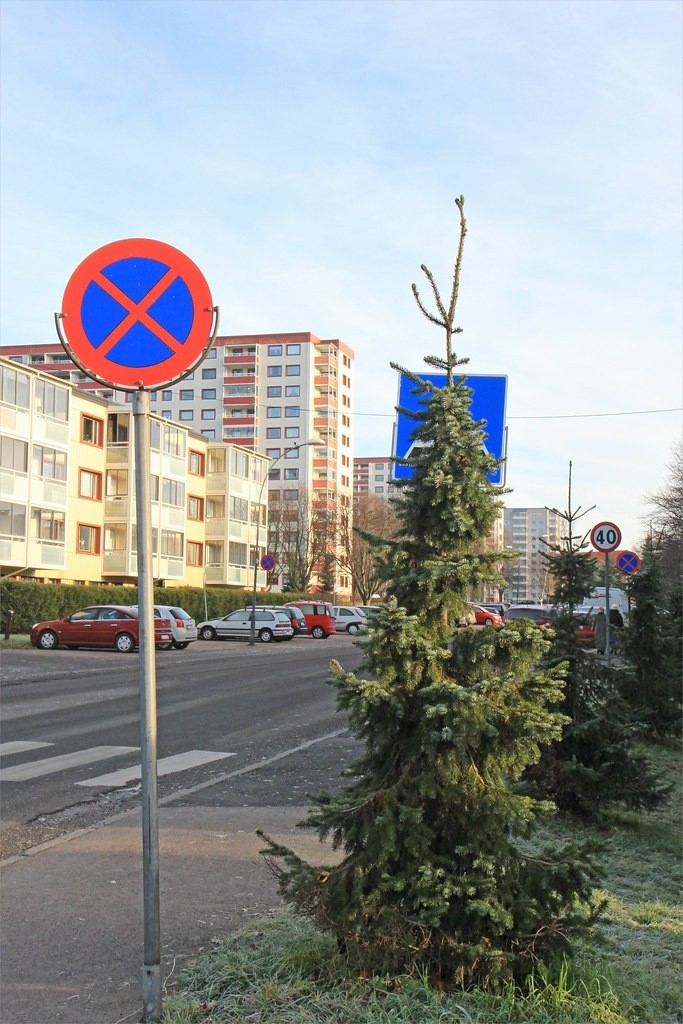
top-left (516, 534), bottom-right (550, 605)
top-left (248, 437), bottom-right (326, 647)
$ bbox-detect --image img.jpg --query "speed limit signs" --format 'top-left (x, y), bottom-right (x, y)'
top-left (590, 521), bottom-right (622, 553)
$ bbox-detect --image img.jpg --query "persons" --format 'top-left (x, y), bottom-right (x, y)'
top-left (610, 605), bottom-right (623, 655)
top-left (591, 607), bottom-right (607, 655)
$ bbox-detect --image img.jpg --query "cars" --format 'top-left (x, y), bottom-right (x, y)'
top-left (332, 606), bottom-right (369, 635)
top-left (282, 599), bottom-right (337, 639)
top-left (209, 602), bottom-right (308, 641)
top-left (108, 603), bottom-right (198, 650)
top-left (29, 604), bottom-right (173, 653)
top-left (356, 605), bottom-right (382, 627)
top-left (195, 607), bottom-right (295, 643)
top-left (446, 585), bottom-right (637, 643)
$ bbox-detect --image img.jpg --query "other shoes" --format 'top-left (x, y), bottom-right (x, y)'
top-left (614, 651), bottom-right (617, 655)
top-left (610, 650), bottom-right (613, 654)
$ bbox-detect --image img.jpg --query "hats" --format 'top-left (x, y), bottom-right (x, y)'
top-left (598, 607), bottom-right (604, 612)
top-left (612, 604), bottom-right (618, 609)
